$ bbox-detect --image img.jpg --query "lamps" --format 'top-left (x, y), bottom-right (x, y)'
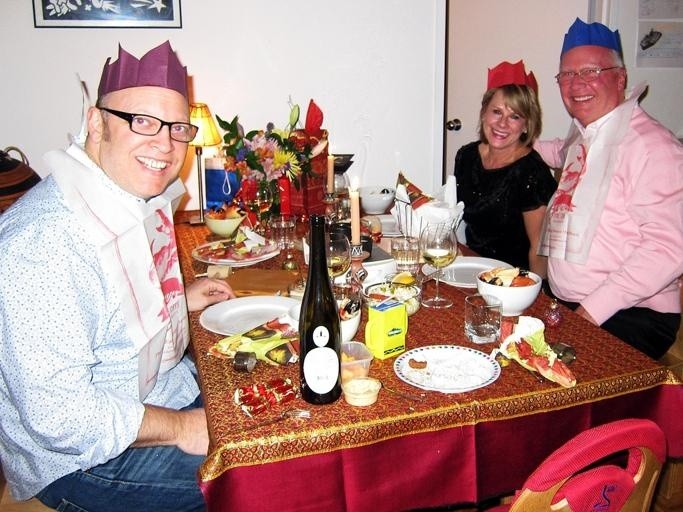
top-left (187, 103), bottom-right (222, 225)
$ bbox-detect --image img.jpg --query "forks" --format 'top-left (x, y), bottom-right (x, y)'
top-left (245, 408), bottom-right (311, 431)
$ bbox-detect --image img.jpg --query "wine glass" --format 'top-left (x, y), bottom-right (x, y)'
top-left (420, 225), bottom-right (458, 309)
top-left (325, 232), bottom-right (352, 288)
top-left (244, 183), bottom-right (273, 236)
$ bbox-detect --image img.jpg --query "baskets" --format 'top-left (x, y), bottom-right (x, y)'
top-left (391, 194), bottom-right (463, 254)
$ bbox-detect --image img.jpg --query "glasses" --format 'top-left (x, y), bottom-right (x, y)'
top-left (554, 66), bottom-right (619, 86)
top-left (98, 107), bottom-right (198, 143)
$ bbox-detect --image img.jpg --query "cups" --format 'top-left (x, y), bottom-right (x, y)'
top-left (330, 224), bottom-right (351, 241)
top-left (269, 213), bottom-right (297, 251)
top-left (301, 236), bottom-right (312, 266)
top-left (391, 235), bottom-right (421, 273)
top-left (464, 294), bottom-right (503, 345)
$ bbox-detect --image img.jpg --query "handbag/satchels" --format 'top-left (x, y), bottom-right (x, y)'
top-left (277, 129), bottom-right (328, 223)
top-left (204, 157), bottom-right (242, 214)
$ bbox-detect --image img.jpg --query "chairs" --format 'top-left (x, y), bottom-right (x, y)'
top-left (488, 417), bottom-right (668, 512)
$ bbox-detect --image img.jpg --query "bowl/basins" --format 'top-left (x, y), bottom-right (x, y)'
top-left (203, 210), bottom-right (247, 239)
top-left (286, 298), bottom-right (361, 345)
top-left (476, 270), bottom-right (542, 318)
top-left (354, 185), bottom-right (396, 215)
top-left (341, 376), bottom-right (382, 406)
top-left (362, 281), bottom-right (422, 316)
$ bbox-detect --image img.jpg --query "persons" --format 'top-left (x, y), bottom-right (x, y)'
top-left (454, 60), bottom-right (557, 279)
top-left (537, 18), bottom-right (683, 361)
top-left (0, 39), bottom-right (235, 510)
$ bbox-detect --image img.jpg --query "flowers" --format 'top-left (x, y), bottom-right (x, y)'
top-left (215, 94), bottom-right (321, 226)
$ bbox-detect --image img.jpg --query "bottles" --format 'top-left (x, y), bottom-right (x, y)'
top-left (281, 252), bottom-right (298, 270)
top-left (360, 231), bottom-right (373, 260)
top-left (298, 215), bottom-right (342, 405)
top-left (543, 298), bottom-right (563, 329)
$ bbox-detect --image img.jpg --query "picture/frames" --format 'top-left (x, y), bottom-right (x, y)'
top-left (32, 0), bottom-right (183, 29)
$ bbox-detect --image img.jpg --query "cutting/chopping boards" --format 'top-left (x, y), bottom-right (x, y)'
top-left (218, 268), bottom-right (307, 298)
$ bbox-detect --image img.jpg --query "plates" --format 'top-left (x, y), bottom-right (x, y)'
top-left (191, 237), bottom-right (281, 268)
top-left (198, 295), bottom-right (301, 339)
top-left (422, 256), bottom-right (515, 288)
top-left (360, 214), bottom-right (404, 237)
top-left (392, 345), bottom-right (501, 394)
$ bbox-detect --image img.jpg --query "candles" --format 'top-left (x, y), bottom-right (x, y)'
top-left (349, 176), bottom-right (361, 245)
top-left (327, 144), bottom-right (334, 193)
